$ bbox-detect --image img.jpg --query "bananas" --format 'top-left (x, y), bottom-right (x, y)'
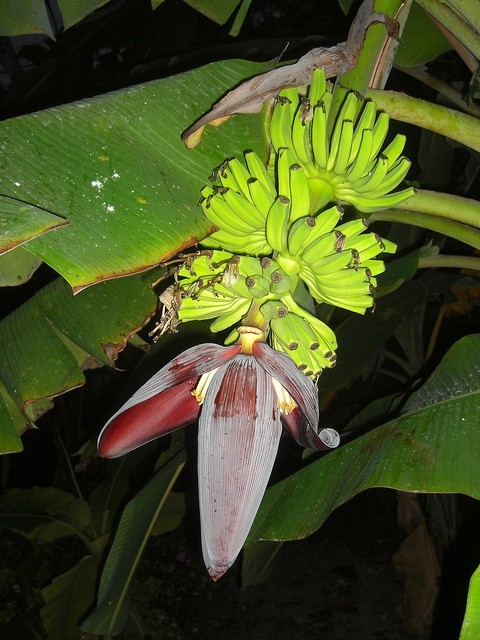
top-left (169, 248), bottom-right (339, 383)
top-left (197, 146), bottom-right (398, 318)
top-left (268, 63), bottom-right (416, 213)
